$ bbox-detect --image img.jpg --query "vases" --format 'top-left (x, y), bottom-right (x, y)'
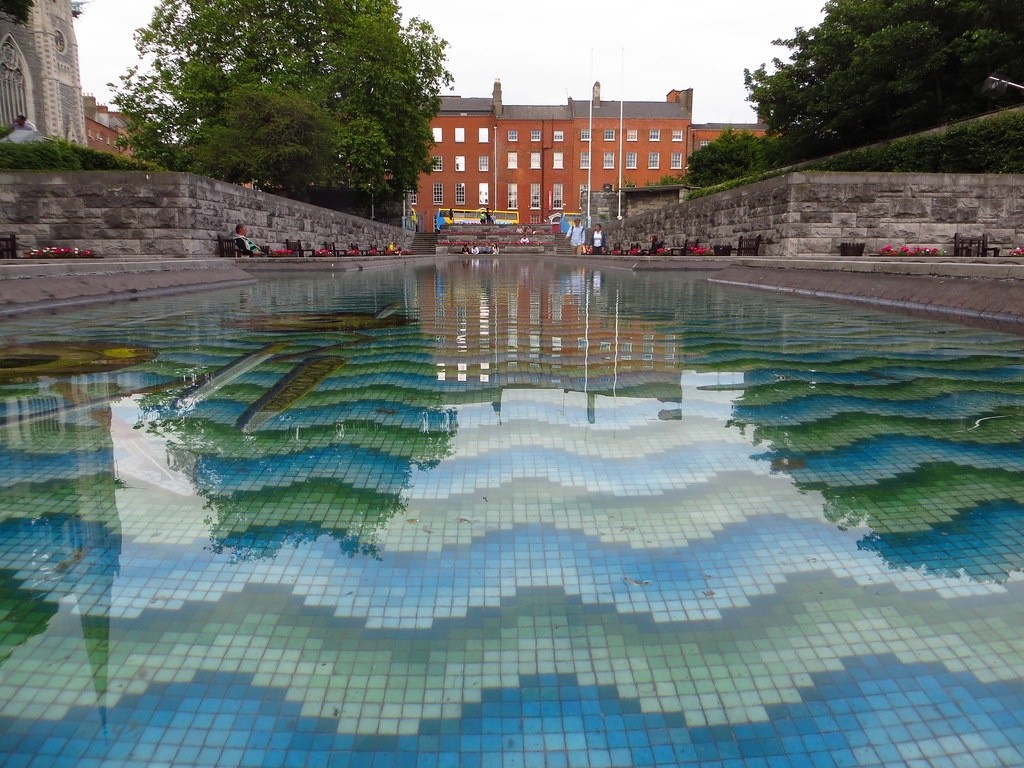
top-left (713, 245), bottom-right (732, 256)
top-left (840, 242), bottom-right (865, 256)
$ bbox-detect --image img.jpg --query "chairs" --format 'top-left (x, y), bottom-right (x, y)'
top-left (284, 239), bottom-right (315, 257)
top-left (217, 234), bottom-right (269, 258)
top-left (670, 238), bottom-right (699, 256)
top-left (621, 242), bottom-right (639, 256)
top-left (0, 234), bottom-right (16, 259)
top-left (726, 234), bottom-right (761, 256)
top-left (386, 246), bottom-right (402, 255)
top-left (324, 241), bottom-right (347, 257)
top-left (351, 242), bottom-right (370, 256)
top-left (642, 241), bottom-right (664, 256)
top-left (954, 233), bottom-right (1001, 257)
top-left (606, 243), bottom-right (621, 255)
top-left (371, 244), bottom-right (385, 256)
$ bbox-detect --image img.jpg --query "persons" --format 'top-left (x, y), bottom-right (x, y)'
top-left (388, 208), bottom-right (530, 255)
top-left (564, 218), bottom-right (658, 256)
top-left (235, 223), bottom-right (266, 258)
top-left (12, 115), bottom-right (32, 131)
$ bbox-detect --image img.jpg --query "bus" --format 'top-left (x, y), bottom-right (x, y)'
top-left (560, 212), bottom-right (582, 233)
top-left (434, 208), bottom-right (519, 231)
